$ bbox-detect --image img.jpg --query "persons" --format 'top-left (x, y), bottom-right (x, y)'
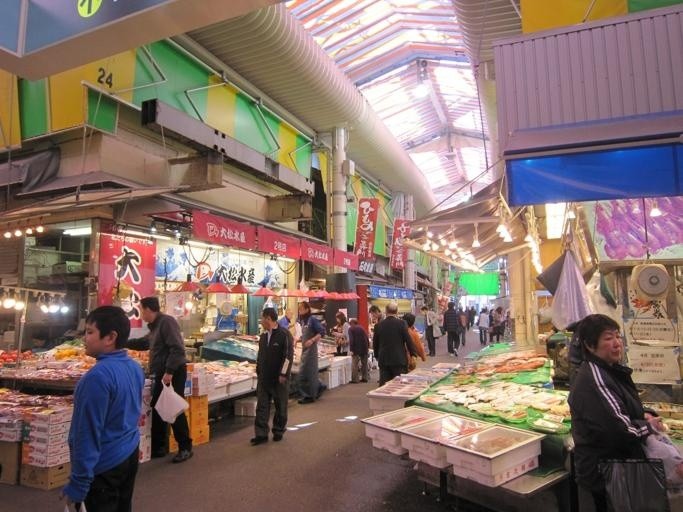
top-left (400, 313), bottom-right (426, 372)
top-left (125, 298), bottom-right (194, 463)
top-left (421, 307), bottom-right (442, 357)
top-left (488, 309), bottom-right (496, 342)
top-left (442, 302), bottom-right (462, 357)
top-left (28, 334), bottom-right (55, 352)
top-left (495, 307), bottom-right (503, 342)
top-left (331, 313), bottom-right (350, 355)
top-left (465, 307), bottom-right (471, 329)
top-left (457, 305), bottom-right (468, 345)
top-left (469, 305), bottom-right (477, 326)
top-left (368, 306), bottom-right (386, 322)
top-left (248, 309), bottom-right (294, 443)
top-left (289, 301), bottom-right (327, 405)
top-left (569, 315), bottom-right (666, 511)
top-left (348, 319), bottom-right (370, 383)
top-left (373, 303), bottom-right (416, 388)
top-left (61, 306), bottom-right (144, 512)
top-left (477, 309), bottom-right (489, 345)
top-left (278, 311), bottom-right (298, 335)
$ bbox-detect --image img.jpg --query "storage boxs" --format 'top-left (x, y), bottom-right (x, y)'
top-left (188, 425), bottom-right (209, 446)
top-left (140, 390), bottom-right (153, 412)
top-left (169, 413), bottom-right (190, 435)
top-left (442, 424), bottom-right (546, 488)
top-left (138, 437), bottom-right (155, 463)
top-left (0, 419), bottom-right (22, 442)
top-left (227, 377), bottom-right (254, 394)
top-left (215, 385), bottom-right (227, 398)
top-left (20, 407), bottom-right (72, 467)
top-left (402, 414), bottom-right (492, 466)
top-left (190, 395), bottom-right (208, 409)
top-left (20, 463), bottom-right (76, 490)
top-left (185, 347), bottom-right (197, 362)
top-left (139, 410), bottom-right (153, 436)
top-left (0, 442), bottom-right (20, 485)
top-left (168, 436), bottom-right (192, 451)
top-left (191, 410), bottom-right (208, 425)
top-left (234, 397), bottom-right (257, 416)
top-left (367, 376), bottom-right (440, 415)
top-left (362, 407), bottom-right (447, 456)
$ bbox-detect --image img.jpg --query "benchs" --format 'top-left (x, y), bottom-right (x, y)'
top-left (413, 461), bottom-right (451, 501)
top-left (445, 465), bottom-right (570, 512)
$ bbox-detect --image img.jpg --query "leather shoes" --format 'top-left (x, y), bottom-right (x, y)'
top-left (299, 397), bottom-right (313, 404)
top-left (273, 433), bottom-right (282, 442)
top-left (251, 435), bottom-right (268, 444)
top-left (316, 384), bottom-right (326, 399)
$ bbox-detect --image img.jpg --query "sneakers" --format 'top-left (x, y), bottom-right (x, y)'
top-left (173, 449), bottom-right (193, 463)
